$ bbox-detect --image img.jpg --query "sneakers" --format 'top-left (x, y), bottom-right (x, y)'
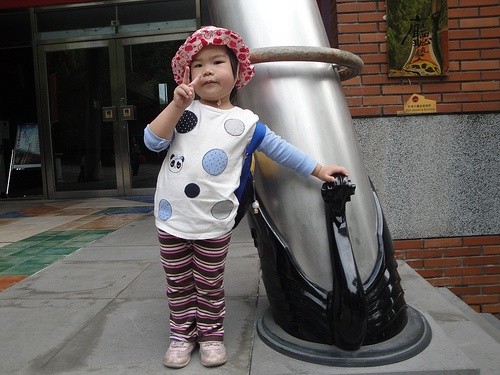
top-left (198, 341), bottom-right (227, 367)
top-left (163, 340), bottom-right (195, 368)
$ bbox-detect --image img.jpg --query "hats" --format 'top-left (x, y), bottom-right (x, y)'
top-left (171, 26), bottom-right (255, 88)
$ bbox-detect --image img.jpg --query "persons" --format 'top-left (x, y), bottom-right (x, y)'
top-left (143, 26), bottom-right (350, 371)
